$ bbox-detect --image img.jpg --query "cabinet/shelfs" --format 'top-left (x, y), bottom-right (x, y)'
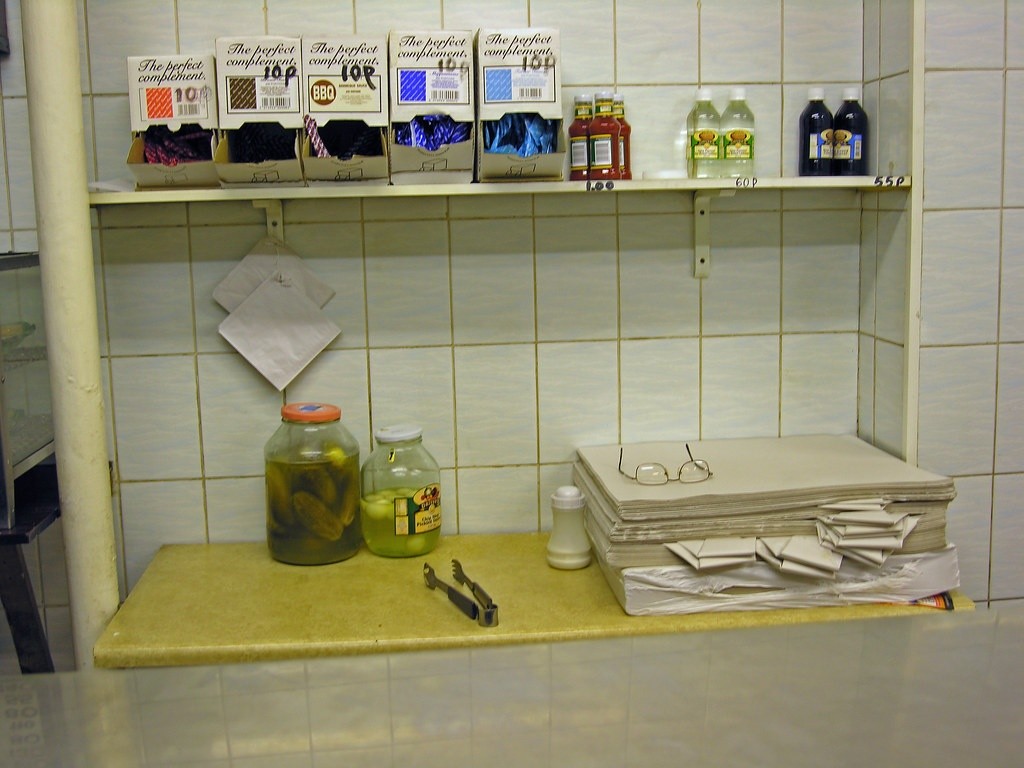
top-left (93, 0), bottom-right (975, 676)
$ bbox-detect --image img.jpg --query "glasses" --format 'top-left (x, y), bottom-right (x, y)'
top-left (619, 444), bottom-right (713, 485)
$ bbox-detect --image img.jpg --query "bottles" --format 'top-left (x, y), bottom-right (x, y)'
top-left (568, 94), bottom-right (594, 179)
top-left (798, 87), bottom-right (833, 176)
top-left (685, 88), bottom-right (721, 179)
top-left (264, 402), bottom-right (361, 566)
top-left (589, 92), bottom-right (621, 180)
top-left (360, 424), bottom-right (442, 557)
top-left (833, 87), bottom-right (869, 176)
top-left (719, 87), bottom-right (756, 179)
top-left (613, 94), bottom-right (632, 180)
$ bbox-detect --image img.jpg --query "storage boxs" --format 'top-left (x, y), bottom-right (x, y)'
top-left (124, 26), bottom-right (570, 184)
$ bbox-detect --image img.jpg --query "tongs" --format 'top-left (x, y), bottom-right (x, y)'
top-left (423, 558), bottom-right (499, 627)
